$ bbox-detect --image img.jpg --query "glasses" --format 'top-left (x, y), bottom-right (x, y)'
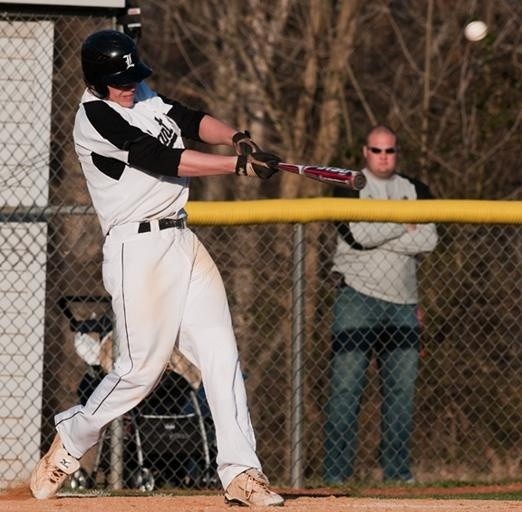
top-left (366, 146), bottom-right (398, 155)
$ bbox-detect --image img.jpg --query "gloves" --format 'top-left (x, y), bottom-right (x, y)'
top-left (234, 151), bottom-right (282, 181)
top-left (231, 128), bottom-right (263, 156)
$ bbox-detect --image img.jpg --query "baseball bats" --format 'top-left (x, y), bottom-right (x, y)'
top-left (278, 163), bottom-right (366, 191)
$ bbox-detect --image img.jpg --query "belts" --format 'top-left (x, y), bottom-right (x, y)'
top-left (137, 217), bottom-right (186, 235)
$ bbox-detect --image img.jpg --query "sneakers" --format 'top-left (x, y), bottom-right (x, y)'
top-left (222, 467), bottom-right (286, 508)
top-left (27, 430), bottom-right (82, 501)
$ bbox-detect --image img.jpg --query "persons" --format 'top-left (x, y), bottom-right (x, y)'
top-left (28, 30), bottom-right (288, 508)
top-left (321, 123), bottom-right (437, 488)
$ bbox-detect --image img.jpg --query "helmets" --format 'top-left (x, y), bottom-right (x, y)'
top-left (80, 29), bottom-right (154, 99)
